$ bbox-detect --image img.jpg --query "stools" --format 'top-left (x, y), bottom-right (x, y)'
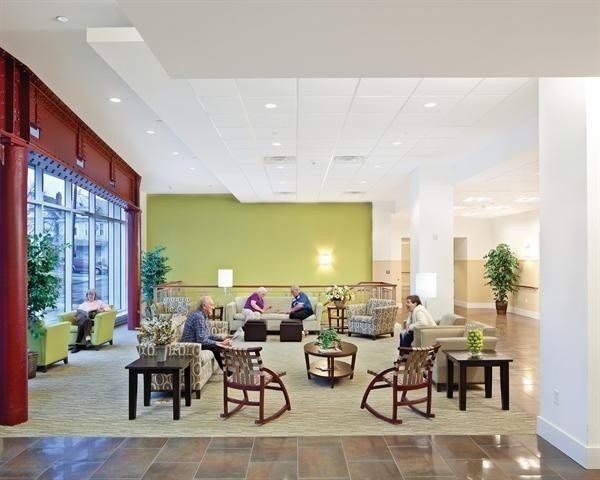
top-left (242, 319), bottom-right (303, 342)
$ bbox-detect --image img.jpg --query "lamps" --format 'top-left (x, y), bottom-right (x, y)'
top-left (415, 272), bottom-right (437, 310)
top-left (217, 268), bottom-right (233, 321)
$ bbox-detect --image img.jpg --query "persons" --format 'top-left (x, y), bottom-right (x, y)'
top-left (70, 289), bottom-right (111, 353)
top-left (392, 295), bottom-right (440, 365)
top-left (178, 295), bottom-right (238, 376)
top-left (285, 285), bottom-right (315, 321)
top-left (242, 287), bottom-right (272, 323)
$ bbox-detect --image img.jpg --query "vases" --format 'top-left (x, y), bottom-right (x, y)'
top-left (155, 344), bottom-right (167, 362)
top-left (334, 299), bottom-right (344, 308)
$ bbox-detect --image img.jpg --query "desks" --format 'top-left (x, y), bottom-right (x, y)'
top-left (124, 355), bottom-right (194, 420)
top-left (442, 349), bottom-right (513, 412)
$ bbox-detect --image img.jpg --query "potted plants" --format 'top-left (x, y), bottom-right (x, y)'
top-left (138, 246), bottom-right (172, 317)
top-left (481, 243), bottom-right (520, 315)
top-left (316, 328), bottom-right (343, 349)
top-left (24, 231), bottom-right (73, 379)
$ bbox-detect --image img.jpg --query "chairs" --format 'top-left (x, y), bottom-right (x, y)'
top-left (26, 318), bottom-right (71, 372)
top-left (145, 296), bottom-right (193, 322)
top-left (56, 304), bottom-right (117, 351)
top-left (215, 342), bottom-right (292, 424)
top-left (345, 298), bottom-right (499, 425)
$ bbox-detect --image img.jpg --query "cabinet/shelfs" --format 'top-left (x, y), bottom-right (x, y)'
top-left (208, 306), bottom-right (224, 321)
top-left (327, 306), bottom-right (348, 334)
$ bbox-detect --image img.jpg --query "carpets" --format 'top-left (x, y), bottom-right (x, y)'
top-left (0, 321), bottom-right (538, 438)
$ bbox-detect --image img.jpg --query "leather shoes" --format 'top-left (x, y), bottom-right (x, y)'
top-left (86, 340), bottom-right (92, 347)
top-left (71, 346), bottom-right (80, 353)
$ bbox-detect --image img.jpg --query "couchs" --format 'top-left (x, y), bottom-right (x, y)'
top-left (226, 296), bottom-right (323, 336)
top-left (136, 321), bottom-right (228, 399)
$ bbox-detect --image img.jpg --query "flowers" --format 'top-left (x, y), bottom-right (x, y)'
top-left (325, 284), bottom-right (352, 301)
top-left (136, 302), bottom-right (184, 346)
